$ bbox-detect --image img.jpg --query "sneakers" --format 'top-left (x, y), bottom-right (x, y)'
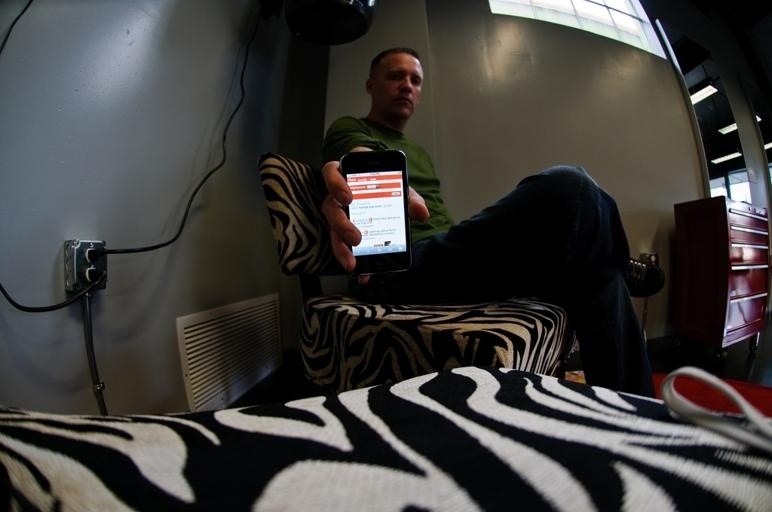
top-left (622, 257), bottom-right (665, 297)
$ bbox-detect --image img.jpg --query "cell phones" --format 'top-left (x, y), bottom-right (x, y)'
top-left (339, 149), bottom-right (413, 277)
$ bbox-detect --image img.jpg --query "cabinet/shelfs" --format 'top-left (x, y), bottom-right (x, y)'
top-left (671, 195), bottom-right (771, 350)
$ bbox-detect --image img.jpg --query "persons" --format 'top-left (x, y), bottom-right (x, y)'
top-left (316, 47), bottom-right (667, 402)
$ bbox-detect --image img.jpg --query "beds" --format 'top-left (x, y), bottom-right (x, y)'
top-left (0, 366), bottom-right (772, 512)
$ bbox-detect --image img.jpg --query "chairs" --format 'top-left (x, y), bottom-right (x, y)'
top-left (258, 154), bottom-right (574, 395)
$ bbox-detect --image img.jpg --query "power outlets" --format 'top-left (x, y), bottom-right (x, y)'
top-left (65, 240), bottom-right (109, 297)
